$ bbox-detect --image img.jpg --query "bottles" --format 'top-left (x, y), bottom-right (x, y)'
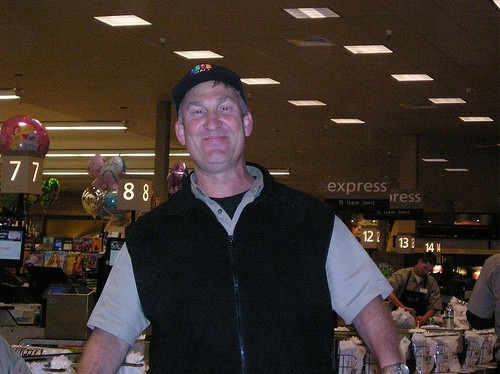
top-left (445, 304), bottom-right (454, 329)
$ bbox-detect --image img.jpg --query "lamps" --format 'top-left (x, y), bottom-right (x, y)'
top-left (42, 120), bottom-right (131, 132)
top-left (0, 88), bottom-right (25, 101)
top-left (43, 148), bottom-right (190, 157)
top-left (39, 167), bottom-right (291, 179)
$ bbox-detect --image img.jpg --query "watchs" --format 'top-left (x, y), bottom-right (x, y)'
top-left (381, 363), bottom-right (409, 374)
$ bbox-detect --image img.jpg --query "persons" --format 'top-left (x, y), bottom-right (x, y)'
top-left (386, 253), bottom-right (442, 323)
top-left (77, 64), bottom-right (409, 374)
top-left (466, 253), bottom-right (500, 374)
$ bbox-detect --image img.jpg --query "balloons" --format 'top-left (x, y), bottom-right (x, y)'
top-left (168, 161), bottom-right (186, 194)
top-left (82, 153), bottom-right (126, 219)
top-left (0, 115), bottom-right (50, 157)
top-left (40, 178), bottom-right (60, 209)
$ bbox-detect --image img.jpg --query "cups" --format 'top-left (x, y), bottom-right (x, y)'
top-left (428, 318), bottom-right (434, 325)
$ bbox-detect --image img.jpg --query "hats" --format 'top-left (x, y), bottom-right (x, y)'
top-left (171, 63), bottom-right (244, 114)
